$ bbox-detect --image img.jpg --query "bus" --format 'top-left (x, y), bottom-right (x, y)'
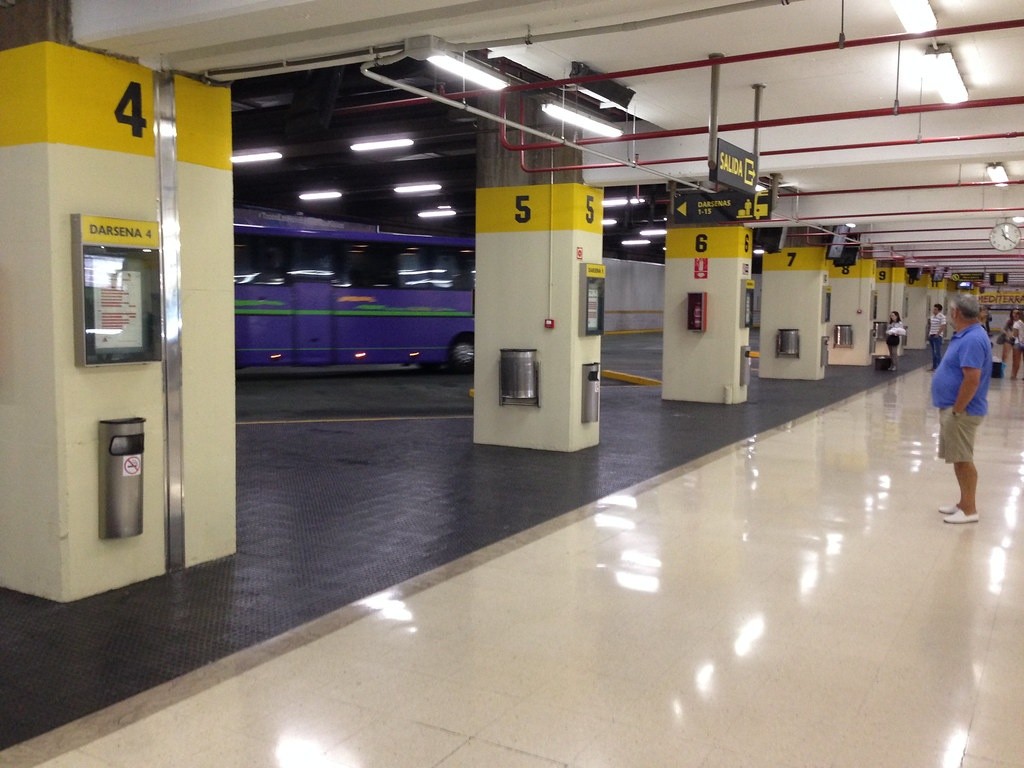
top-left (234, 220), bottom-right (476, 377)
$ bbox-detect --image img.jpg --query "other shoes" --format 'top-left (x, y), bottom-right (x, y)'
top-left (944, 509), bottom-right (980, 524)
top-left (938, 502), bottom-right (959, 515)
top-left (927, 367), bottom-right (935, 372)
top-left (888, 365), bottom-right (893, 371)
top-left (891, 365), bottom-right (897, 372)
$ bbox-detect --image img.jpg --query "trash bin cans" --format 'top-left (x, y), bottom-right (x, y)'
top-left (821, 335), bottom-right (828, 367)
top-left (870, 330), bottom-right (876, 353)
top-left (835, 324), bottom-right (852, 344)
top-left (902, 326), bottom-right (908, 346)
top-left (874, 322), bottom-right (887, 339)
top-left (582, 362), bottom-right (599, 423)
top-left (741, 345), bottom-right (751, 387)
top-left (98, 417), bottom-right (146, 540)
top-left (778, 329), bottom-right (799, 354)
top-left (500, 349), bottom-right (537, 398)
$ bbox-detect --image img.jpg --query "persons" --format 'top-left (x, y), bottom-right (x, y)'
top-left (931, 292), bottom-right (993, 524)
top-left (1000, 308), bottom-right (1024, 381)
top-left (886, 311), bottom-right (903, 371)
top-left (926, 303), bottom-right (947, 372)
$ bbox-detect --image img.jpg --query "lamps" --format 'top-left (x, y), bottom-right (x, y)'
top-left (541, 99), bottom-right (623, 139)
top-left (405, 34), bottom-right (511, 93)
top-left (986, 162), bottom-right (1008, 187)
top-left (892, 1), bottom-right (937, 35)
top-left (924, 45), bottom-right (972, 105)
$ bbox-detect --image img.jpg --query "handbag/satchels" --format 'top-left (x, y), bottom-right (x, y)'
top-left (995, 334), bottom-right (1009, 346)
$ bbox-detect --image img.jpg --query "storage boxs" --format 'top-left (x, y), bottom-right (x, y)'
top-left (875, 358), bottom-right (891, 370)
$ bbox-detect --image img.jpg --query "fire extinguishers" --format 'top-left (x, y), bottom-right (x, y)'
top-left (693, 302), bottom-right (701, 330)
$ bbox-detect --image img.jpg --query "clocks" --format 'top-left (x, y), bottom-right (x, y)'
top-left (989, 223), bottom-right (1021, 251)
